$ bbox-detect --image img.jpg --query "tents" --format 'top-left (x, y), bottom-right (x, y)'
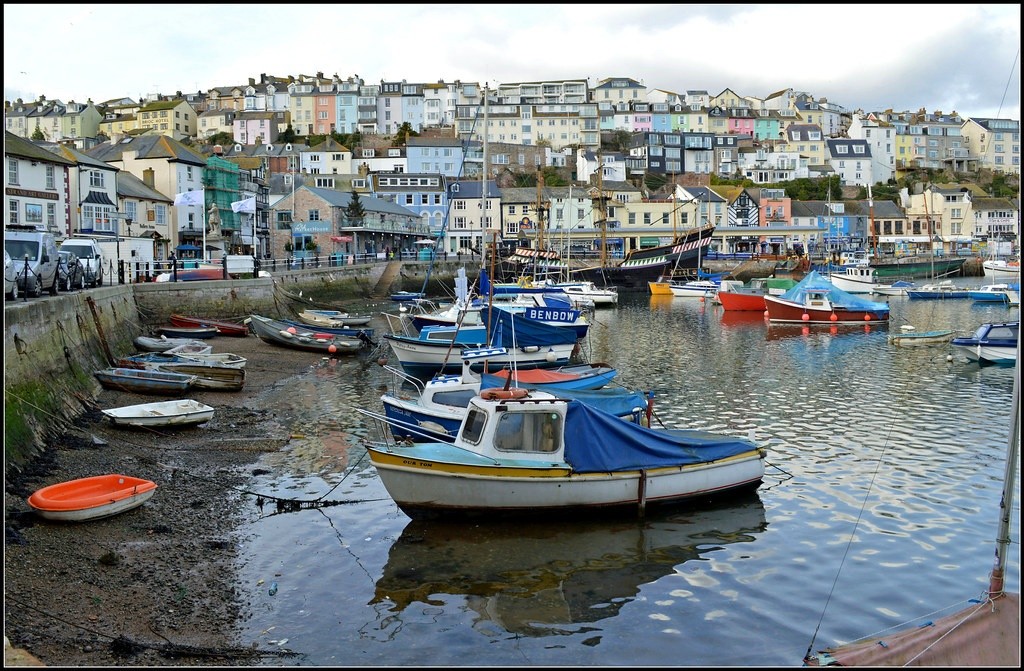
top-left (413, 239), bottom-right (436, 244)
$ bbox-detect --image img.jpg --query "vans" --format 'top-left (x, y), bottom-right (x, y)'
top-left (58, 238), bottom-right (104, 288)
top-left (6, 223), bottom-right (59, 298)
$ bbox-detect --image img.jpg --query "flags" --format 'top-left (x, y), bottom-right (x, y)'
top-left (174, 190), bottom-right (204, 206)
top-left (231, 197), bottom-right (255, 214)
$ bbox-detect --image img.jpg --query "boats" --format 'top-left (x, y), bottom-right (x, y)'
top-left (133, 334), bottom-right (207, 352)
top-left (93, 367), bottom-right (198, 396)
top-left (172, 352), bottom-right (247, 367)
top-left (648, 172), bottom-right (1020, 368)
top-left (100, 399), bottom-right (215, 427)
top-left (356, 232), bottom-right (767, 520)
top-left (169, 313), bottom-right (251, 338)
top-left (299, 309), bottom-right (372, 329)
top-left (113, 351), bottom-right (246, 391)
top-left (163, 345), bottom-right (213, 354)
top-left (28, 475), bottom-right (158, 523)
top-left (250, 314), bottom-right (375, 354)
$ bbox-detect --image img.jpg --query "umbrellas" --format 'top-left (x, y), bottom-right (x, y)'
top-left (199, 245), bottom-right (222, 259)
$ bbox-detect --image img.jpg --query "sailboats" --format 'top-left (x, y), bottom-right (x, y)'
top-left (382, 81), bottom-right (709, 339)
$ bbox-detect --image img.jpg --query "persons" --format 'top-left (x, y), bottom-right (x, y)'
top-left (208, 203), bottom-right (220, 232)
top-left (169, 251), bottom-right (175, 260)
top-left (386, 247), bottom-right (394, 260)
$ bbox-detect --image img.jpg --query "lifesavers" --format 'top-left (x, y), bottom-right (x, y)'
top-left (480, 387), bottom-right (528, 399)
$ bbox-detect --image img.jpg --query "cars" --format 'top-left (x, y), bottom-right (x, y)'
top-left (5, 250), bottom-right (18, 300)
top-left (57, 251), bottom-right (85, 291)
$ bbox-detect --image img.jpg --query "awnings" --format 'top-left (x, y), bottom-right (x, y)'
top-left (595, 239), bottom-right (624, 245)
top-left (174, 244), bottom-right (202, 256)
top-left (640, 237), bottom-right (659, 246)
